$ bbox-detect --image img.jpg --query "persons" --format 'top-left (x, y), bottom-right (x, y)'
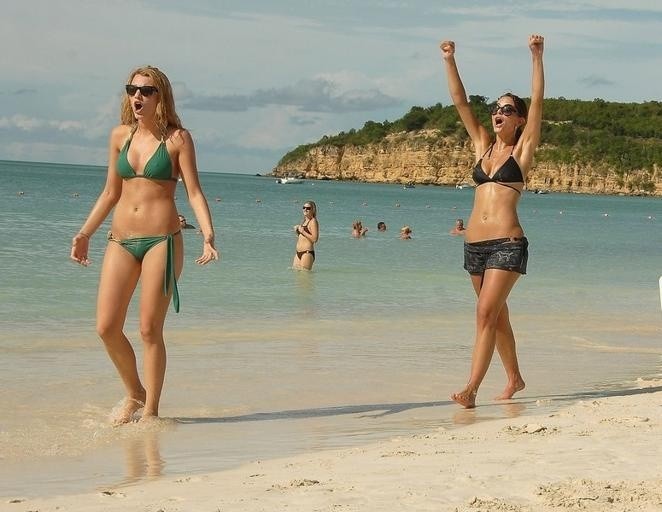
top-left (292, 202), bottom-right (319, 271)
top-left (352, 219), bottom-right (368, 238)
top-left (441, 34), bottom-right (544, 409)
top-left (378, 222), bottom-right (386, 232)
top-left (449, 219), bottom-right (466, 234)
top-left (178, 215), bottom-right (195, 229)
top-left (69, 65), bottom-right (219, 426)
top-left (399, 226), bottom-right (412, 240)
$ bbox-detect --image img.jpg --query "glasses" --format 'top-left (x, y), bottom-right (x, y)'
top-left (303, 206), bottom-right (311, 210)
top-left (488, 103), bottom-right (516, 116)
top-left (125, 85), bottom-right (158, 97)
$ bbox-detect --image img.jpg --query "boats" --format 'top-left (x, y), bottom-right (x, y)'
top-left (276, 177), bottom-right (300, 184)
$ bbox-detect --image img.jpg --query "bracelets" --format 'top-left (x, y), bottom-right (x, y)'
top-left (78, 232), bottom-right (90, 240)
